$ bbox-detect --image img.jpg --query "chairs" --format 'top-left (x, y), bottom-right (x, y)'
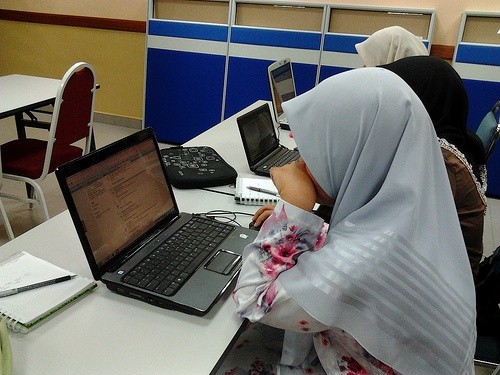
top-left (0, 62), bottom-right (97, 220)
top-left (476, 101), bottom-right (500, 161)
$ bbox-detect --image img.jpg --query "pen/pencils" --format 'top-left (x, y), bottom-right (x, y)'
top-left (247, 186), bottom-right (276, 196)
top-left (0, 274), bottom-right (78, 298)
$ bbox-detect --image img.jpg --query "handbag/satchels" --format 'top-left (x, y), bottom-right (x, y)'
top-left (159, 146), bottom-right (237, 190)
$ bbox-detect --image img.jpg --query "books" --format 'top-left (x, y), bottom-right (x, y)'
top-left (0, 249), bottom-right (98, 333)
top-left (235, 178), bottom-right (320, 211)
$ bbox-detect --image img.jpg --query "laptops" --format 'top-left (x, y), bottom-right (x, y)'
top-left (236, 102), bottom-right (301, 177)
top-left (56, 126), bottom-right (259, 317)
top-left (268, 58), bottom-right (297, 130)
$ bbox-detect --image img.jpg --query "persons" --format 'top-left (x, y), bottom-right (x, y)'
top-left (375, 56), bottom-right (488, 277)
top-left (233, 67), bottom-right (477, 375)
top-left (354, 25), bottom-right (429, 67)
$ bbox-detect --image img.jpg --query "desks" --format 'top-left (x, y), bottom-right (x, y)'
top-left (0, 190), bottom-right (263, 375)
top-left (181, 100), bottom-right (321, 211)
top-left (0, 73), bottom-right (100, 208)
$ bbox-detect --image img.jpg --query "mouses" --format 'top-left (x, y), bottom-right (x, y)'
top-left (249, 219), bottom-right (262, 231)
